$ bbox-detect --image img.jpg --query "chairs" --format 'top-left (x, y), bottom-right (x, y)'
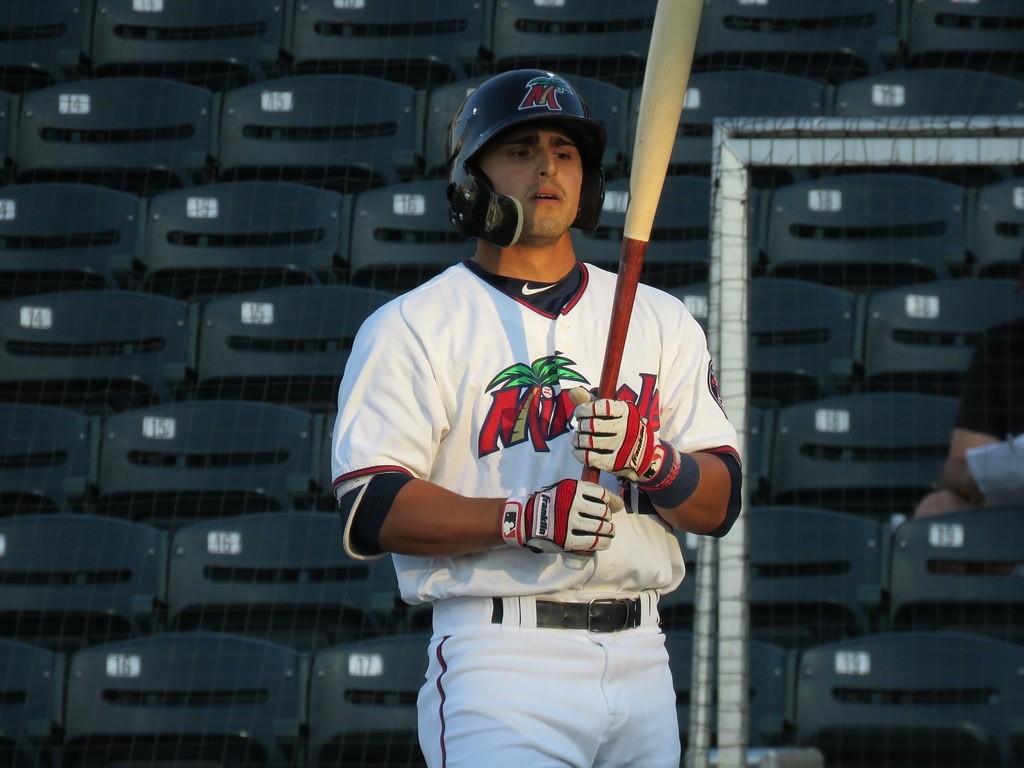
top-left (795, 632), bottom-right (1024, 768)
top-left (0, 1), bottom-right (1024, 642)
top-left (306, 632), bottom-right (432, 768)
top-left (67, 631), bottom-right (309, 767)
top-left (659, 630), bottom-right (795, 750)
top-left (0, 643), bottom-right (66, 768)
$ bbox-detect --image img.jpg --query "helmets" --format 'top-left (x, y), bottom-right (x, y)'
top-left (446, 69), bottom-right (608, 249)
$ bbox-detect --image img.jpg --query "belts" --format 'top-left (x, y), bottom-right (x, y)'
top-left (492, 597), bottom-right (642, 634)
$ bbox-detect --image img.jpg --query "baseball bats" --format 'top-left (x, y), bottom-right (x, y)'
top-left (562, 1), bottom-right (705, 571)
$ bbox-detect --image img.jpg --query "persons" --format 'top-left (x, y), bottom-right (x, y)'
top-left (331, 70), bottom-right (748, 768)
top-left (913, 315), bottom-right (1024, 518)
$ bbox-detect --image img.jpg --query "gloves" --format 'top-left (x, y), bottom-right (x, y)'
top-left (500, 478), bottom-right (625, 555)
top-left (568, 387), bottom-right (700, 510)
